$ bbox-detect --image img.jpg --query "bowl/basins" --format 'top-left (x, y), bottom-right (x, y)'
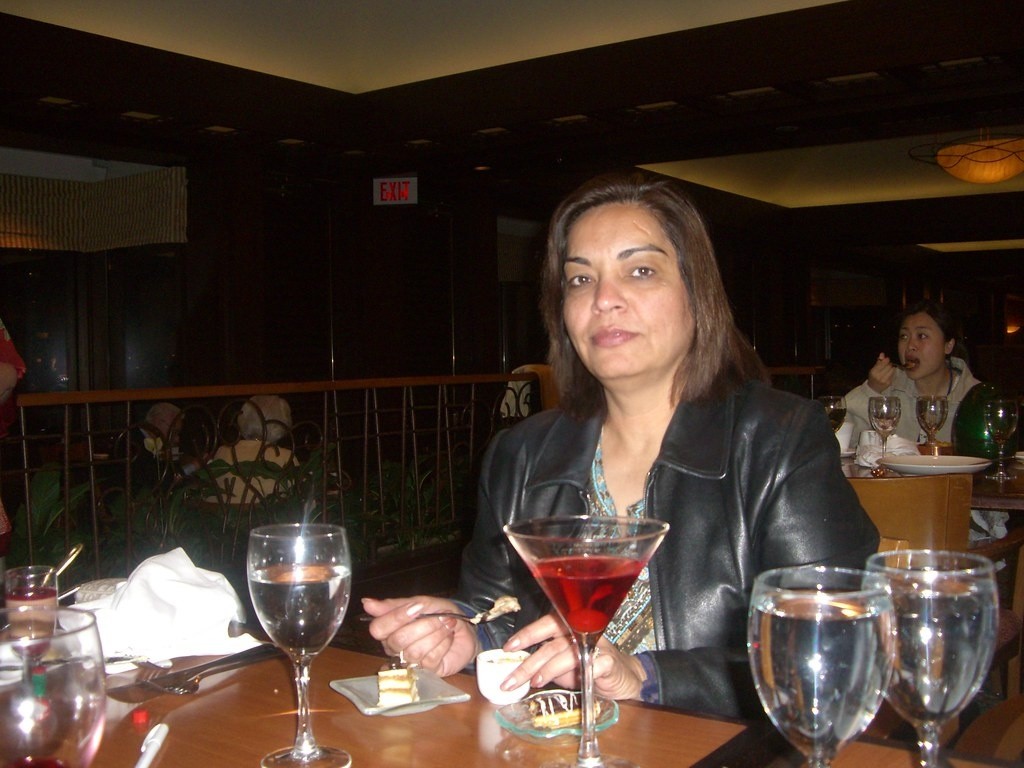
top-left (495, 689), bottom-right (618, 746)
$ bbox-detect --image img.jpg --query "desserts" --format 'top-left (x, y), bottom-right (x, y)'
top-left (376, 650), bottom-right (420, 706)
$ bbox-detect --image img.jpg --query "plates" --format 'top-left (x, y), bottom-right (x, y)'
top-left (1, 644), bottom-right (63, 686)
top-left (330, 669), bottom-right (470, 717)
top-left (876, 455), bottom-right (992, 476)
top-left (840, 449), bottom-right (856, 457)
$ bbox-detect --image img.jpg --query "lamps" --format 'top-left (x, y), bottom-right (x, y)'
top-left (908, 132), bottom-right (1024, 185)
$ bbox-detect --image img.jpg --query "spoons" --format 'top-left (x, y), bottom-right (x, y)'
top-left (878, 358), bottom-right (911, 369)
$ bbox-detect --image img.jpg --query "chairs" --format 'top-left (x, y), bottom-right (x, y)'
top-left (846, 473), bottom-right (1024, 763)
top-left (190, 496), bottom-right (295, 566)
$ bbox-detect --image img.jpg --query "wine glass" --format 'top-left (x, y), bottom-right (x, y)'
top-left (747, 564), bottom-right (896, 768)
top-left (916, 396), bottom-right (948, 446)
top-left (4, 565), bottom-right (58, 696)
top-left (983, 398), bottom-right (1019, 479)
top-left (503, 514), bottom-right (671, 768)
top-left (862, 550), bottom-right (998, 768)
top-left (246, 523), bottom-right (351, 768)
top-left (868, 396), bottom-right (901, 458)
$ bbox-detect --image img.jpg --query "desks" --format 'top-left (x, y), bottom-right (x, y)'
top-left (0, 603), bottom-right (1024, 768)
top-left (840, 446), bottom-right (1024, 509)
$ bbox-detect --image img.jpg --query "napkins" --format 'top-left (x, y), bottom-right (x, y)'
top-left (58, 545), bottom-right (262, 665)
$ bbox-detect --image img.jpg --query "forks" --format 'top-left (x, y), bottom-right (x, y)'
top-left (131, 660), bottom-right (173, 670)
top-left (360, 599), bottom-right (512, 622)
top-left (163, 650), bottom-right (285, 695)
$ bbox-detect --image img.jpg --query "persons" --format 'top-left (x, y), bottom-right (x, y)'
top-left (0, 321), bottom-right (27, 399)
top-left (136, 395), bottom-right (302, 504)
top-left (835, 297), bottom-right (979, 451)
top-left (360, 172), bottom-right (881, 725)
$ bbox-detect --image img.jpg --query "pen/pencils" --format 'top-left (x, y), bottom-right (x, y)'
top-left (134, 723), bottom-right (170, 768)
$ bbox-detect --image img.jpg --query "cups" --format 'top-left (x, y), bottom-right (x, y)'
top-left (860, 430), bottom-right (883, 446)
top-left (835, 423), bottom-right (854, 452)
top-left (1, 607), bottom-right (107, 768)
top-left (477, 649), bottom-right (532, 705)
top-left (817, 396), bottom-right (847, 433)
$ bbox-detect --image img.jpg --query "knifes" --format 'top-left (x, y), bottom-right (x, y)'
top-left (105, 643), bottom-right (276, 703)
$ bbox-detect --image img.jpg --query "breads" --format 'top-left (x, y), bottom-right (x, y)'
top-left (528, 695), bottom-right (601, 729)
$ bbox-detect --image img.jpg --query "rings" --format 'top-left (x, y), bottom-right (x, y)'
top-left (400, 651), bottom-right (410, 666)
top-left (594, 647), bottom-right (601, 659)
